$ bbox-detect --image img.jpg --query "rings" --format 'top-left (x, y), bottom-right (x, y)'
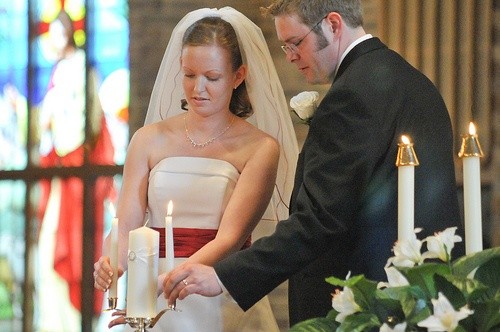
top-left (182, 279), bottom-right (188, 286)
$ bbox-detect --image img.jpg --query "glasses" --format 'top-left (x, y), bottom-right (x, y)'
top-left (281, 15), bottom-right (327, 54)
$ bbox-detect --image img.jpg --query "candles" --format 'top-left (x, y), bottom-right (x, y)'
top-left (396, 135), bottom-right (419, 243)
top-left (126, 220), bottom-right (159, 320)
top-left (165, 200), bottom-right (174, 275)
top-left (109, 202), bottom-right (118, 299)
top-left (459, 123), bottom-right (484, 255)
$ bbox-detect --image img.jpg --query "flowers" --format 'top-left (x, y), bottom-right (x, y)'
top-left (289, 227), bottom-right (499, 332)
top-left (289, 91), bottom-right (321, 128)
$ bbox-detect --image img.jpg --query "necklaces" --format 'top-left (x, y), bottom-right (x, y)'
top-left (184, 112), bottom-right (236, 148)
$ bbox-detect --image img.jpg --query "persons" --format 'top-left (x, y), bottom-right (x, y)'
top-left (91, 5), bottom-right (299, 332)
top-left (161, 0), bottom-right (466, 332)
